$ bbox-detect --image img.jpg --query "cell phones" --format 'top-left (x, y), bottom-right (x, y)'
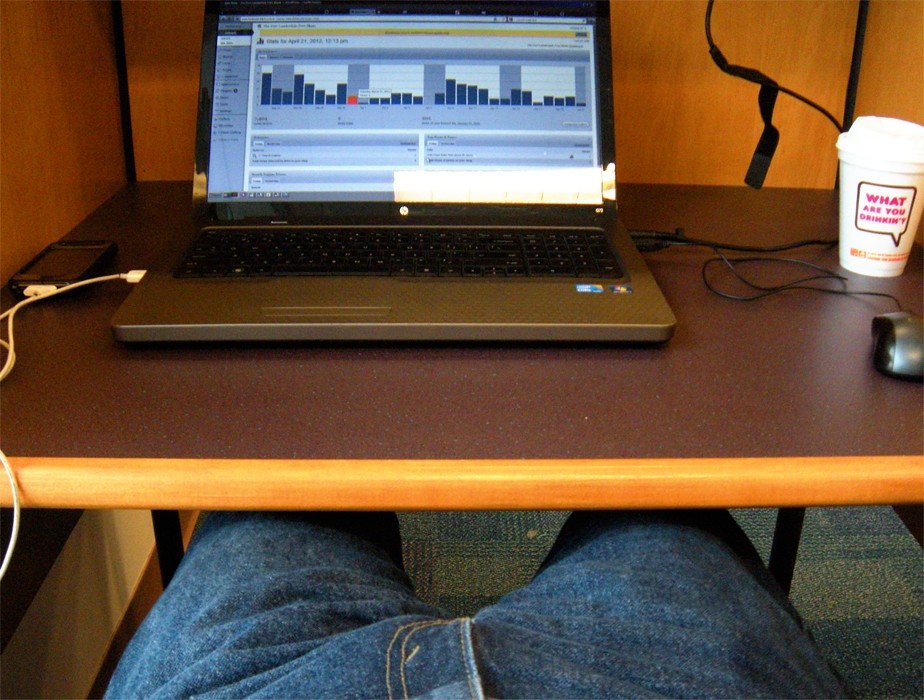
top-left (7, 239), bottom-right (119, 300)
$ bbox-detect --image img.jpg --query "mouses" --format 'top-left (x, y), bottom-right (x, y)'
top-left (871, 309), bottom-right (924, 382)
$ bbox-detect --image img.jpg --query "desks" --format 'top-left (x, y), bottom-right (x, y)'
top-left (0, 180), bottom-right (924, 593)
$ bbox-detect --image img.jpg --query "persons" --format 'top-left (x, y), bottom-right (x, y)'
top-left (100, 507), bottom-right (847, 700)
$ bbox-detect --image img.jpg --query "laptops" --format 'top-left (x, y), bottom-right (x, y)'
top-left (109, 1), bottom-right (678, 349)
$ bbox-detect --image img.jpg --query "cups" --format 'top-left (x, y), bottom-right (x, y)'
top-left (834, 116), bottom-right (924, 278)
